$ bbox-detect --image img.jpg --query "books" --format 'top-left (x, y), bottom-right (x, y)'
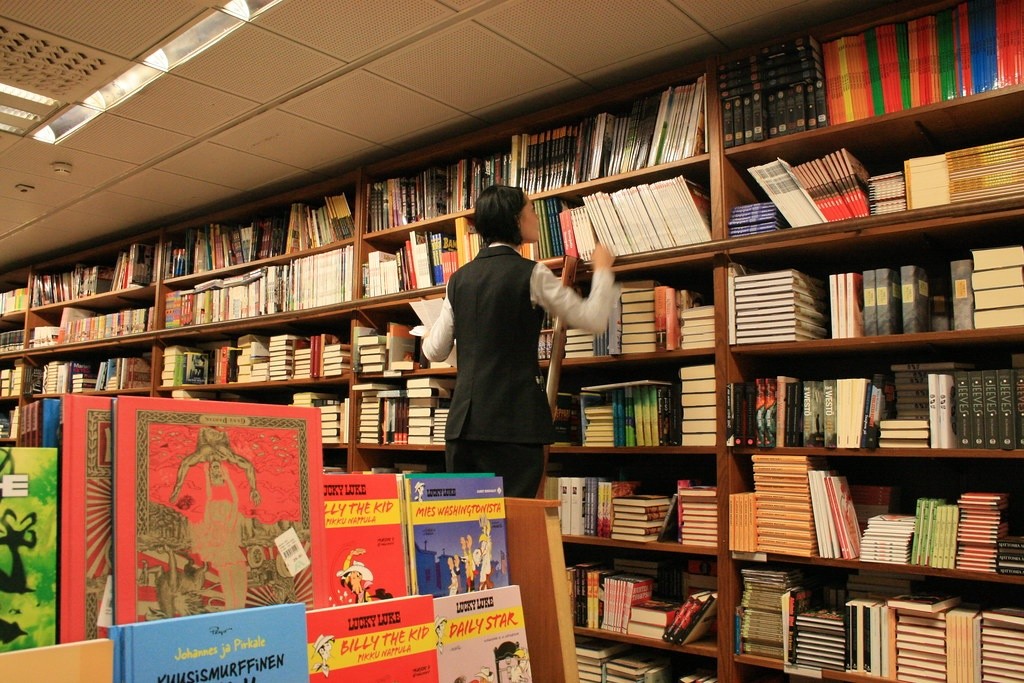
top-left (122, 601), bottom-right (310, 682)
top-left (305, 592), bottom-right (439, 683)
top-left (0, 638), bottom-right (114, 683)
top-left (108, 623), bottom-right (127, 683)
top-left (513, 0), bottom-right (1024, 681)
top-left (432, 584), bottom-right (533, 683)
top-left (0, 152), bottom-right (514, 651)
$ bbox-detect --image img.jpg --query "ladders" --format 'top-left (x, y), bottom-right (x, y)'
top-left (534, 256), bottom-right (579, 498)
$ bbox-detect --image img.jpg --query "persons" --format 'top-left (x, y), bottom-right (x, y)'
top-left (419, 184), bottom-right (615, 500)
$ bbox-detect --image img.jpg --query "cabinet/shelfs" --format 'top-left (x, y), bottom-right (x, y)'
top-left (0, 0), bottom-right (1024, 683)
